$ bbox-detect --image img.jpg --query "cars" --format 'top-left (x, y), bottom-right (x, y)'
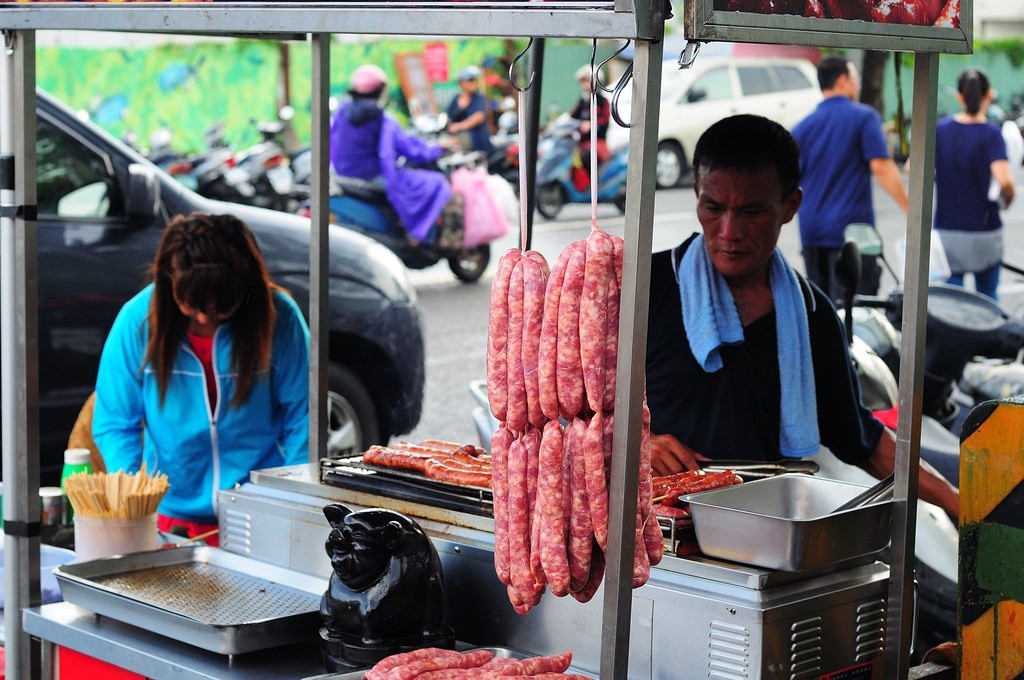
top-left (0, 87), bottom-right (425, 487)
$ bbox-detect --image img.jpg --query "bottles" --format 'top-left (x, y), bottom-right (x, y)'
top-left (60, 447), bottom-right (94, 528)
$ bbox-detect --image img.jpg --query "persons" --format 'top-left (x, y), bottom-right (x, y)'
top-left (793, 56), bottom-right (908, 310)
top-left (901, 68), bottom-right (1016, 299)
top-left (650, 114), bottom-right (958, 528)
top-left (482, 38), bottom-right (522, 116)
top-left (334, 64), bottom-right (453, 266)
top-left (574, 63), bottom-right (610, 176)
top-left (90, 211), bottom-right (310, 545)
top-left (445, 66), bottom-right (492, 150)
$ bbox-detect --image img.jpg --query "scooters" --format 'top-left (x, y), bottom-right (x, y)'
top-left (835, 223), bottom-right (1024, 489)
top-left (122, 96), bottom-right (541, 283)
top-left (535, 111), bottom-right (629, 219)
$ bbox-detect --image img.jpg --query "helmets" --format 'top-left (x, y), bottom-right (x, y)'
top-left (577, 64), bottom-right (606, 94)
top-left (455, 65), bottom-right (481, 82)
top-left (348, 64), bottom-right (386, 95)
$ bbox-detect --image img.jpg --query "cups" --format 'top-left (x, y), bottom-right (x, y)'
top-left (73, 511), bottom-right (158, 563)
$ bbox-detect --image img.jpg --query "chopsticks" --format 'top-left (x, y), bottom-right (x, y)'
top-left (64, 461), bottom-right (169, 519)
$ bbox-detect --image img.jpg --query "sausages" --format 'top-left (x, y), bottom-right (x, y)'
top-left (485, 228), bottom-right (663, 616)
top-left (363, 440), bottom-right (494, 490)
top-left (363, 648), bottom-right (596, 680)
top-left (651, 467), bottom-right (743, 526)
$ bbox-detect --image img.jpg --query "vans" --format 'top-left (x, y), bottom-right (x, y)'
top-left (543, 58), bottom-right (825, 190)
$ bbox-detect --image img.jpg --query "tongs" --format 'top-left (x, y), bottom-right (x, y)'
top-left (827, 471), bottom-right (895, 518)
top-left (696, 458), bottom-right (820, 479)
